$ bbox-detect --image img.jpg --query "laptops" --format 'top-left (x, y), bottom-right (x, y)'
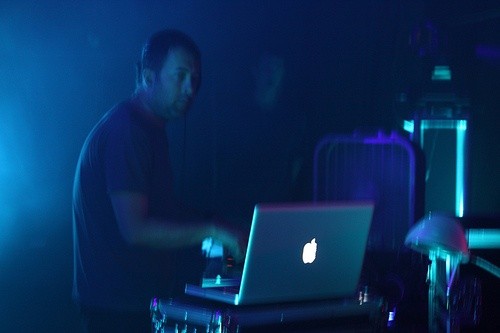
top-left (184, 201), bottom-right (375, 307)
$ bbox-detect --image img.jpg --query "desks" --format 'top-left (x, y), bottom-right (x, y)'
top-left (150, 293), bottom-right (383, 333)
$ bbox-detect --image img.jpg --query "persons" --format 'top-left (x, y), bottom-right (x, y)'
top-left (72, 28), bottom-right (248, 333)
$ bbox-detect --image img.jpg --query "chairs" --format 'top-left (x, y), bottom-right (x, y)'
top-left (314, 135), bottom-right (415, 287)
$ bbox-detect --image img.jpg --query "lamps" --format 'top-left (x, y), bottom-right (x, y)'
top-left (404, 212), bottom-right (500, 278)
